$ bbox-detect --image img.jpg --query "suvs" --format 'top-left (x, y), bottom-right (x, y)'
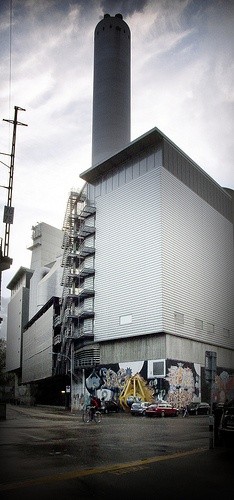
top-left (183, 401), bottom-right (211, 416)
top-left (131, 402), bottom-right (150, 416)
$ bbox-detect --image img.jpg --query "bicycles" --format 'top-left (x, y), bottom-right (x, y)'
top-left (82, 404), bottom-right (103, 423)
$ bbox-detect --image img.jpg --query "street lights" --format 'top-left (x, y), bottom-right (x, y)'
top-left (47, 352), bottom-right (74, 414)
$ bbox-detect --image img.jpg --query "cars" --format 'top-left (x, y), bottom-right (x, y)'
top-left (209, 402), bottom-right (234, 447)
top-left (146, 403), bottom-right (180, 417)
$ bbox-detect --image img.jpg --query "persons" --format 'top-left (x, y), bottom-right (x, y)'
top-left (183, 404), bottom-right (190, 418)
top-left (88, 395), bottom-right (98, 422)
top-left (93, 396), bottom-right (102, 412)
